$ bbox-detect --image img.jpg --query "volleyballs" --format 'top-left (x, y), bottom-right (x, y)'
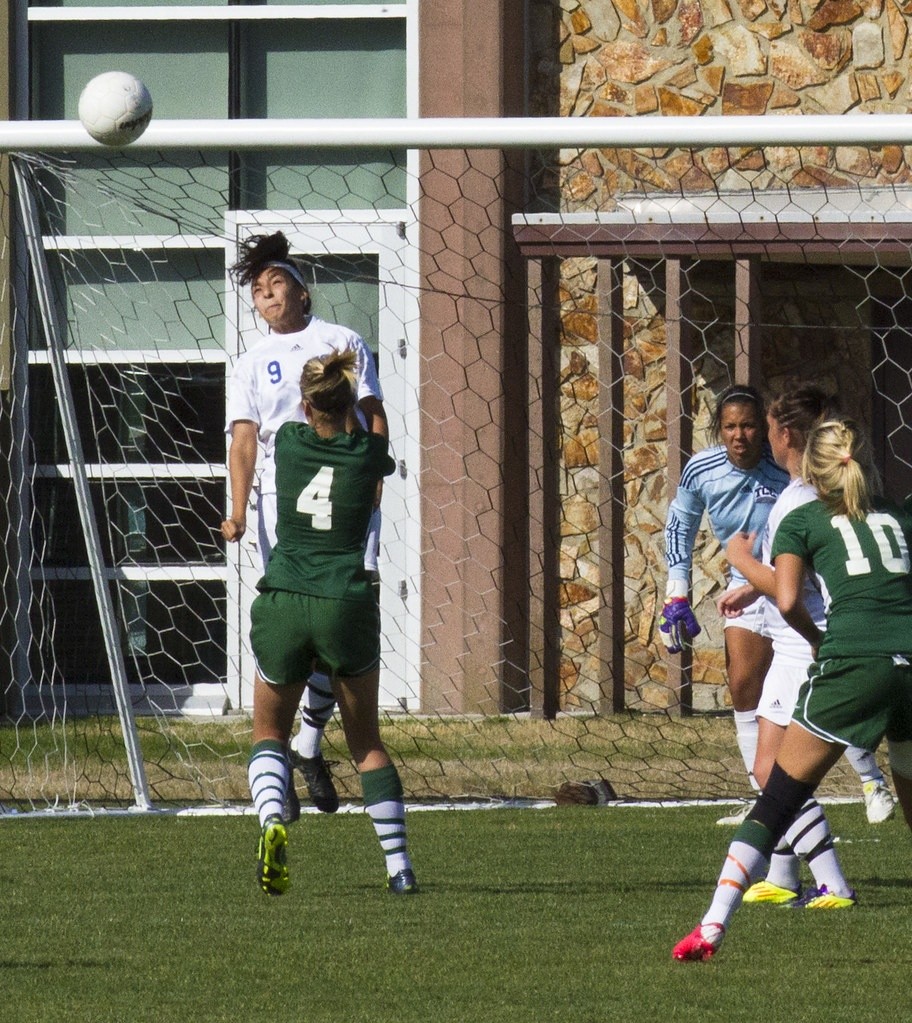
top-left (76, 71), bottom-right (154, 148)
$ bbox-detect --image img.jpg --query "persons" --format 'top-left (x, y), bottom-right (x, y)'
top-left (716, 378), bottom-right (859, 911)
top-left (673, 416), bottom-right (912, 961)
top-left (219, 257), bottom-right (391, 827)
top-left (656, 384), bottom-right (897, 827)
top-left (247, 347), bottom-right (419, 897)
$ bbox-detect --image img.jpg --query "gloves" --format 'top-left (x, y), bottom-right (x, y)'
top-left (657, 579), bottom-right (701, 654)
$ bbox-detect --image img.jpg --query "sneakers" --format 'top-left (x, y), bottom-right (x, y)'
top-left (741, 879), bottom-right (802, 906)
top-left (256, 817), bottom-right (289, 898)
top-left (792, 885), bottom-right (857, 907)
top-left (281, 766), bottom-right (301, 824)
top-left (715, 803), bottom-right (754, 825)
top-left (671, 923), bottom-right (727, 961)
top-left (288, 740), bottom-right (339, 812)
top-left (385, 869), bottom-right (419, 895)
top-left (863, 780), bottom-right (895, 824)
